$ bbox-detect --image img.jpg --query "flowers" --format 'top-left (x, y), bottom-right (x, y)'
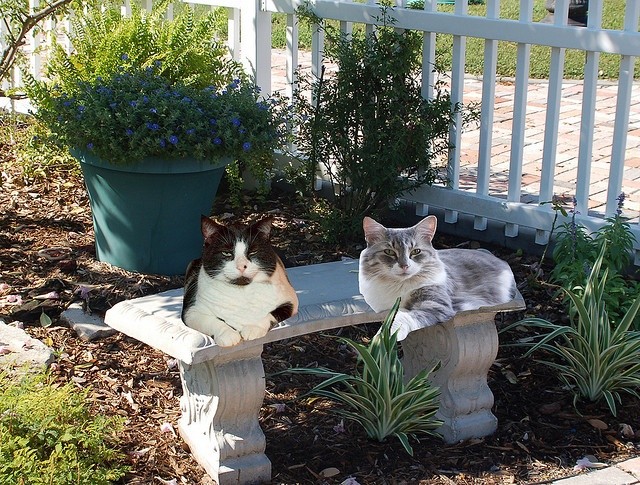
top-left (54, 51), bottom-right (264, 157)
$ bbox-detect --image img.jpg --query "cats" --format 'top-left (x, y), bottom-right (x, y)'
top-left (180, 212), bottom-right (300, 348)
top-left (357, 214), bottom-right (518, 342)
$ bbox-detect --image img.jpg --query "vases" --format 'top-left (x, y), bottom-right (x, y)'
top-left (66, 147), bottom-right (233, 276)
